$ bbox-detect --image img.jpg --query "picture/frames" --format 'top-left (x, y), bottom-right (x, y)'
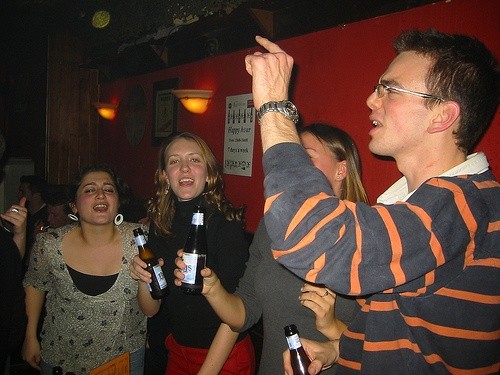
top-left (151, 78), bottom-right (179, 144)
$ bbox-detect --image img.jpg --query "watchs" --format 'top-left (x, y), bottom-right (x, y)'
top-left (256, 101), bottom-right (299, 127)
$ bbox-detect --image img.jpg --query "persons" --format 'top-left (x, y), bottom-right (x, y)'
top-left (175, 124), bottom-right (371, 375)
top-left (244, 29), bottom-right (500, 375)
top-left (129, 130), bottom-right (250, 375)
top-left (19, 172), bottom-right (143, 236)
top-left (0, 137), bottom-right (28, 375)
top-left (23, 167), bottom-right (160, 375)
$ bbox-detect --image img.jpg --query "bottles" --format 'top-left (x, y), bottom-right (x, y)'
top-left (180, 201), bottom-right (208, 295)
top-left (284, 324), bottom-right (312, 375)
top-left (132, 227), bottom-right (170, 300)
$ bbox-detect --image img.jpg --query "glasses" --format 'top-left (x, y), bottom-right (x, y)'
top-left (372, 83), bottom-right (446, 104)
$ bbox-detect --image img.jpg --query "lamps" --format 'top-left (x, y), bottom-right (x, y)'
top-left (95, 104), bottom-right (115, 120)
top-left (172, 90), bottom-right (212, 114)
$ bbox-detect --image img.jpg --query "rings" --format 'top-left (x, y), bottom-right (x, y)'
top-left (321, 289), bottom-right (331, 298)
top-left (12, 208), bottom-right (18, 214)
